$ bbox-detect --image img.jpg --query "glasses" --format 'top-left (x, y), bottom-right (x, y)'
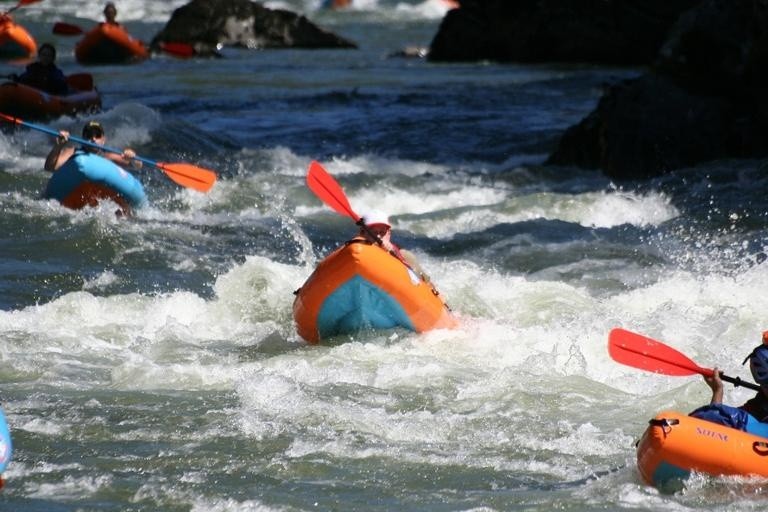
top-left (86, 132), bottom-right (102, 139)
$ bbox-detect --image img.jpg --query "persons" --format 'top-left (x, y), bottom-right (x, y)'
top-left (352, 209), bottom-right (429, 282)
top-left (99, 3), bottom-right (120, 27)
top-left (45, 120), bottom-right (142, 172)
top-left (703, 343), bottom-right (768, 424)
top-left (15, 42), bottom-right (68, 95)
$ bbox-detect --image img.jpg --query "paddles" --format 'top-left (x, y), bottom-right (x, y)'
top-left (51, 20), bottom-right (192, 59)
top-left (0, 0), bottom-right (43, 15)
top-left (0, 113), bottom-right (217, 193)
top-left (306, 158), bottom-right (453, 310)
top-left (608, 327), bottom-right (767, 396)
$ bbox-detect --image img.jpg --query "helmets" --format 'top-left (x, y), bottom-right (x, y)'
top-left (750, 344), bottom-right (768, 384)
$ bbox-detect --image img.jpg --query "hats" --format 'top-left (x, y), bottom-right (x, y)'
top-left (359, 210), bottom-right (392, 228)
top-left (83, 121), bottom-right (104, 135)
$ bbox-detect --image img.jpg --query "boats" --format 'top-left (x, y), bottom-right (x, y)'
top-left (0, 73), bottom-right (102, 127)
top-left (41, 149), bottom-right (150, 216)
top-left (635, 406), bottom-right (768, 494)
top-left (291, 235), bottom-right (459, 345)
top-left (0, 20), bottom-right (37, 64)
top-left (74, 22), bottom-right (151, 66)
